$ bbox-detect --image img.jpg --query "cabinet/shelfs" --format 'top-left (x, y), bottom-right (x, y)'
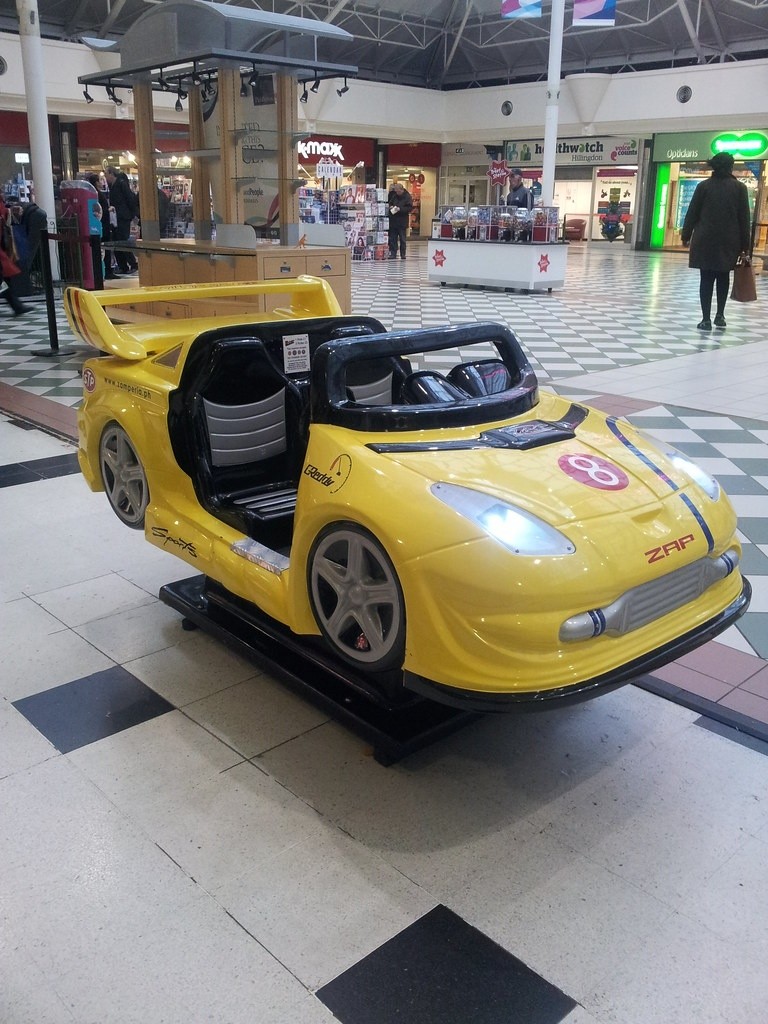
top-left (299, 188), bottom-right (403, 262)
top-left (123, 165), bottom-right (218, 242)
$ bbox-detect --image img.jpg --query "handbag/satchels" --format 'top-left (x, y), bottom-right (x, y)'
top-left (731, 253), bottom-right (758, 301)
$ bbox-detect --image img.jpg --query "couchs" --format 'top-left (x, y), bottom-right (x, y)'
top-left (563, 218), bottom-right (586, 243)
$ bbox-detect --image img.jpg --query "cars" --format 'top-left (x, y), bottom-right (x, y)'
top-left (62, 275), bottom-right (754, 714)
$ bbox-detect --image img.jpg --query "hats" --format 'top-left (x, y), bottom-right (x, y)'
top-left (511, 168), bottom-right (523, 177)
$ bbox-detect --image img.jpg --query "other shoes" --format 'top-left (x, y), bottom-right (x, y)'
top-left (388, 253), bottom-right (396, 259)
top-left (116, 267), bottom-right (129, 274)
top-left (15, 306), bottom-right (34, 314)
top-left (401, 255), bottom-right (406, 259)
top-left (105, 273), bottom-right (121, 279)
top-left (127, 266), bottom-right (137, 274)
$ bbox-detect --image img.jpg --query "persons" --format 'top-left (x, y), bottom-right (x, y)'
top-left (507, 143), bottom-right (531, 161)
top-left (104, 166), bottom-right (138, 274)
top-left (345, 188), bottom-right (354, 203)
top-left (88, 173), bottom-right (122, 279)
top-left (503, 168), bottom-right (534, 242)
top-left (136, 179), bottom-right (169, 240)
top-left (388, 183), bottom-right (413, 260)
top-left (0, 189), bottom-right (34, 314)
top-left (680, 152), bottom-right (750, 330)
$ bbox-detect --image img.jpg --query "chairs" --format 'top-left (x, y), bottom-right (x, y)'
top-left (327, 327), bottom-right (423, 408)
top-left (194, 337), bottom-right (320, 538)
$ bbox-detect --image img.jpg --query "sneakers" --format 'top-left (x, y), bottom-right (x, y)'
top-left (697, 319), bottom-right (712, 329)
top-left (714, 313), bottom-right (727, 326)
